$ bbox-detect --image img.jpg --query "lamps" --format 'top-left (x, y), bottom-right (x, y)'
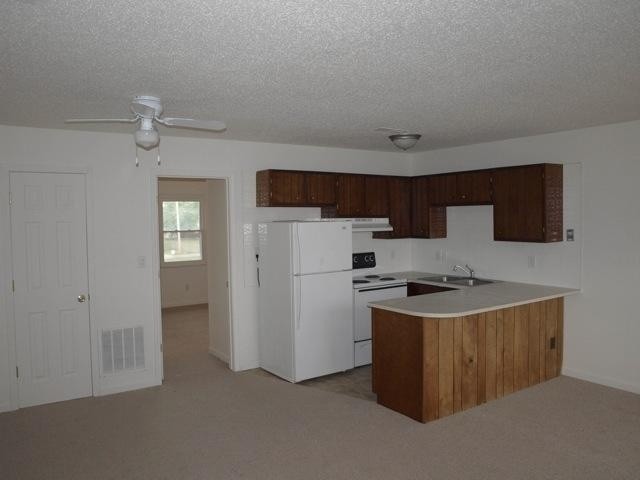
top-left (389, 134), bottom-right (421, 151)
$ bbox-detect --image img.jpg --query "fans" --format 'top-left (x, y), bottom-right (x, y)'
top-left (64, 95), bottom-right (227, 168)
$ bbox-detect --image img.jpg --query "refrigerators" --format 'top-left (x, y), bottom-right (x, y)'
top-left (258, 220), bottom-right (355, 384)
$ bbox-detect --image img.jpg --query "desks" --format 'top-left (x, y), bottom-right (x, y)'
top-left (367, 284), bottom-right (582, 424)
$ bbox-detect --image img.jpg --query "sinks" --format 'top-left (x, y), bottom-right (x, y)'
top-left (417, 275), bottom-right (493, 287)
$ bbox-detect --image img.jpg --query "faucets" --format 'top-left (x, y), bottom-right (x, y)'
top-left (453, 264), bottom-right (474, 278)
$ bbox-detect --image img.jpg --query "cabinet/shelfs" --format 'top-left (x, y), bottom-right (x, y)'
top-left (493, 162), bottom-right (563, 243)
top-left (321, 172), bottom-right (366, 218)
top-left (256, 169), bottom-right (338, 206)
top-left (430, 169), bottom-right (493, 205)
top-left (366, 175), bottom-right (412, 240)
top-left (412, 176), bottom-right (448, 239)
